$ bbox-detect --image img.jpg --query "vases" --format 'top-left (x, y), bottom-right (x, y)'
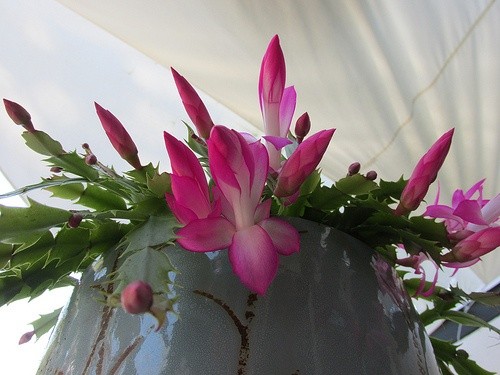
top-left (34, 217), bottom-right (444, 375)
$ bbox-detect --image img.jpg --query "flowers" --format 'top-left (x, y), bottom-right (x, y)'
top-left (0, 33), bottom-right (500, 375)
